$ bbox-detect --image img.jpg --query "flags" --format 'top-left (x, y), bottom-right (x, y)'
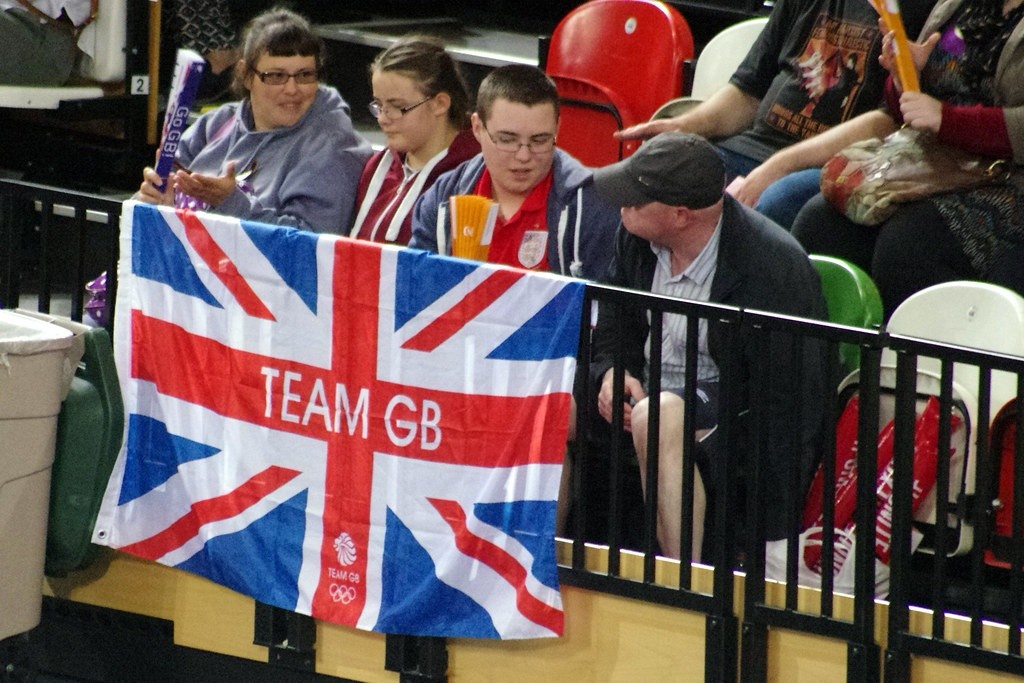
top-left (88, 198), bottom-right (584, 644)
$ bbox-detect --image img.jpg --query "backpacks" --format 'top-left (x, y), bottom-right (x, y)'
top-left (85, 181), bottom-right (257, 327)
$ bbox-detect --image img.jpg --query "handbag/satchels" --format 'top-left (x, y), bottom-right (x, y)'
top-left (765, 527), bottom-right (891, 599)
top-left (820, 123), bottom-right (1011, 225)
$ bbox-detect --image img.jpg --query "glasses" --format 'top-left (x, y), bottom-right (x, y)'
top-left (368, 96), bottom-right (433, 120)
top-left (250, 65), bottom-right (319, 85)
top-left (482, 120), bottom-right (556, 154)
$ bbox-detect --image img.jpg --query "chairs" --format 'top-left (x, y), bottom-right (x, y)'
top-left (546, 1), bottom-right (695, 172)
top-left (0, 0), bottom-right (128, 109)
top-left (806, 254), bottom-right (882, 385)
top-left (643, 17), bottom-right (770, 150)
top-left (838, 278), bottom-right (1024, 557)
top-left (982, 396), bottom-right (1024, 569)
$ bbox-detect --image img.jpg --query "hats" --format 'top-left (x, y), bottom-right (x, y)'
top-left (593, 133), bottom-right (728, 209)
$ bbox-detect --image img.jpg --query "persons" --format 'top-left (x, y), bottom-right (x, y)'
top-left (343, 33), bottom-right (485, 249)
top-left (0, 0), bottom-right (250, 105)
top-left (613, 0), bottom-right (1024, 333)
top-left (404, 63), bottom-right (625, 399)
top-left (554, 132), bottom-right (838, 565)
top-left (128, 9), bottom-right (376, 237)
